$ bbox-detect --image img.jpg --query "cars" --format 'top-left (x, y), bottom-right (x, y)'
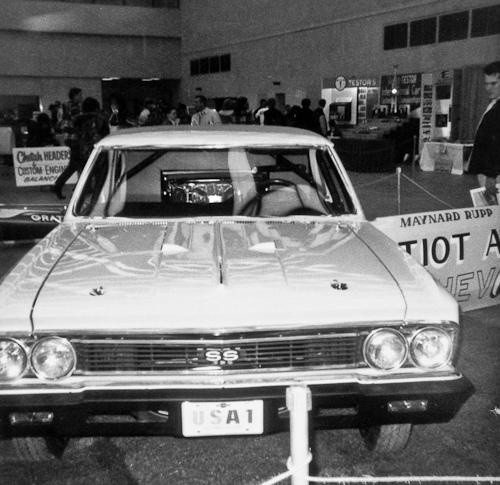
top-left (0, 123), bottom-right (470, 465)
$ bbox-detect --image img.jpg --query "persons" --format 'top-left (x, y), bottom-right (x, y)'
top-left (464, 60), bottom-right (499, 207)
top-left (13, 87), bottom-right (109, 197)
top-left (109, 95), bottom-right (222, 129)
top-left (231, 95), bottom-right (330, 139)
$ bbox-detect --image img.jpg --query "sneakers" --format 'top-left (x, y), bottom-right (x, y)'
top-left (49, 186), bottom-right (66, 200)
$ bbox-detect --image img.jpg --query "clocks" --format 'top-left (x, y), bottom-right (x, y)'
top-left (334, 75), bottom-right (347, 91)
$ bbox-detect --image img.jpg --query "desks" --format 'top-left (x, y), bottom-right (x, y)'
top-left (418, 141), bottom-right (473, 176)
